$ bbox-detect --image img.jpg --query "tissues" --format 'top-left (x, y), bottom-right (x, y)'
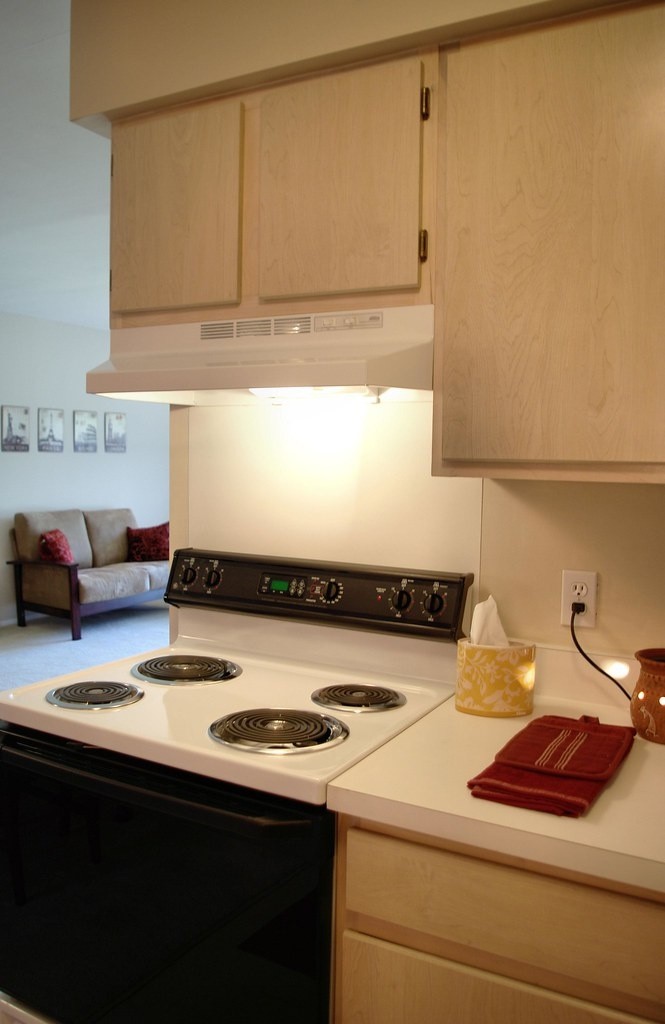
top-left (456, 595), bottom-right (538, 717)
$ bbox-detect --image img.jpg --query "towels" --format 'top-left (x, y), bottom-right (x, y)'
top-left (466, 714), bottom-right (637, 819)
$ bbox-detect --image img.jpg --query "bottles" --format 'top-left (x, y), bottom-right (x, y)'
top-left (631, 648), bottom-right (665, 744)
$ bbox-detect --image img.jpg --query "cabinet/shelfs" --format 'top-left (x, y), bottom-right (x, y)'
top-left (109, 42), bottom-right (429, 328)
top-left (335, 807), bottom-right (665, 1024)
top-left (431, 0), bottom-right (665, 482)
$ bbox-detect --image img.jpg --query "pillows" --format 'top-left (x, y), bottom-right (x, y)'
top-left (36, 528), bottom-right (76, 566)
top-left (127, 518), bottom-right (169, 562)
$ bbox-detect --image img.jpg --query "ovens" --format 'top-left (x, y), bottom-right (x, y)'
top-left (0, 719), bottom-right (336, 1024)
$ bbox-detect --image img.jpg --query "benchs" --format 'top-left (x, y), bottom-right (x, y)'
top-left (8, 508), bottom-right (171, 641)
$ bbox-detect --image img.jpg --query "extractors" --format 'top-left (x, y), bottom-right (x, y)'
top-left (83, 302), bottom-right (435, 407)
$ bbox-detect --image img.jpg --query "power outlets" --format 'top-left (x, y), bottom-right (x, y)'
top-left (560, 569), bottom-right (597, 628)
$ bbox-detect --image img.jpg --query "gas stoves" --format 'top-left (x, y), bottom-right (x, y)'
top-left (0, 548), bottom-right (474, 807)
